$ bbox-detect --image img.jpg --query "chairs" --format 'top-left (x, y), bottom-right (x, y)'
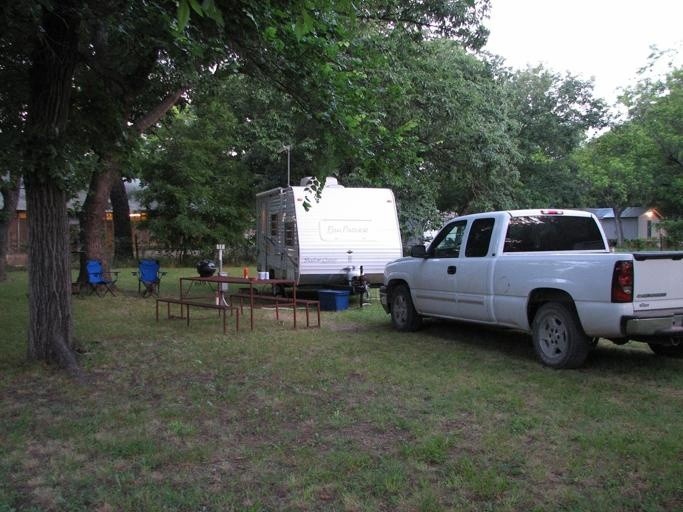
top-left (131, 259), bottom-right (167, 297)
top-left (85, 259), bottom-right (119, 299)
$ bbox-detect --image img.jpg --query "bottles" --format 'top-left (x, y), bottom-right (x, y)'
top-left (244, 268), bottom-right (248, 279)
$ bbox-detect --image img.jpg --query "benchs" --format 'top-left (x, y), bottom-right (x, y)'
top-left (152, 295), bottom-right (240, 334)
top-left (230, 292), bottom-right (321, 329)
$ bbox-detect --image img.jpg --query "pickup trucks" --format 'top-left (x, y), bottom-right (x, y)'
top-left (378, 207), bottom-right (682, 370)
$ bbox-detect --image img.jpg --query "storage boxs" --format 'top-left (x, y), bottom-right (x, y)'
top-left (317, 287), bottom-right (351, 312)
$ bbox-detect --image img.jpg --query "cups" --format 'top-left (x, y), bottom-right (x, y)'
top-left (257, 272), bottom-right (269, 280)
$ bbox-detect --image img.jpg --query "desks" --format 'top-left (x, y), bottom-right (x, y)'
top-left (178, 273), bottom-right (296, 332)
